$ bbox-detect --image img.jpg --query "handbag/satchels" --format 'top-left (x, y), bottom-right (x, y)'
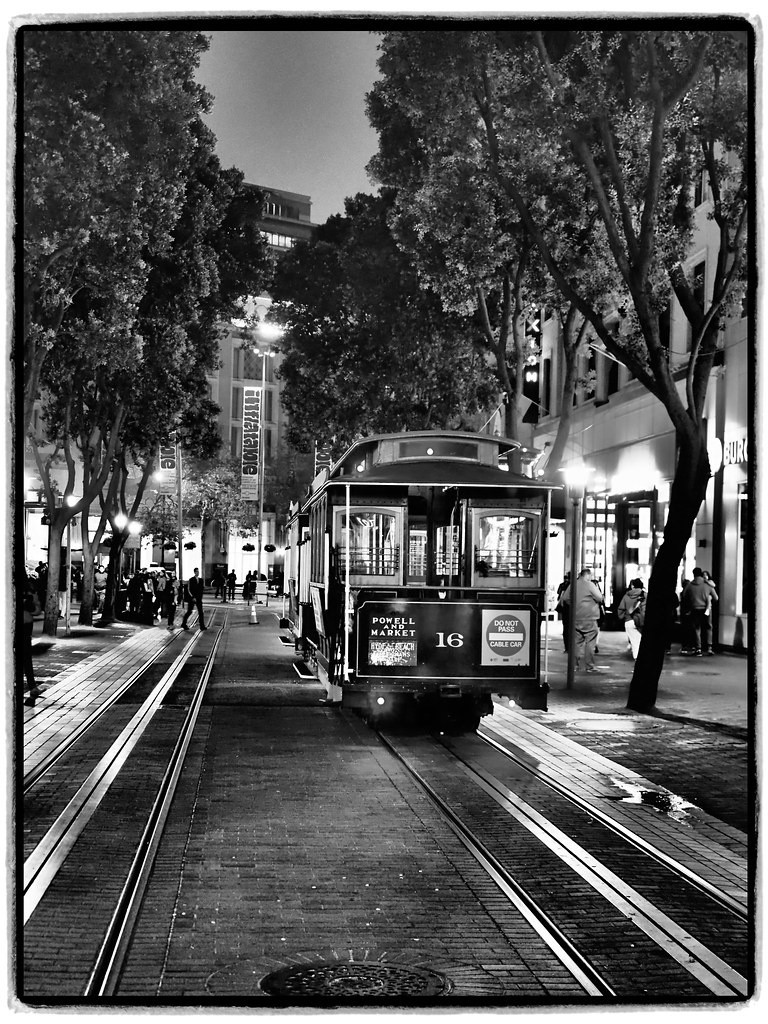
top-left (633, 591), bottom-right (647, 628)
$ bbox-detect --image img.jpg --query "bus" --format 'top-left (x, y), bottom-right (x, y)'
top-left (276, 428), bottom-right (552, 730)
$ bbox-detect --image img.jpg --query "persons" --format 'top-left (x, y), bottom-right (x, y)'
top-left (557, 569), bottom-right (602, 673)
top-left (180, 568), bottom-right (208, 630)
top-left (665, 568), bottom-right (718, 657)
top-left (617, 579), bottom-right (648, 661)
top-left (268, 573), bottom-right (280, 590)
top-left (93, 563), bottom-right (180, 630)
top-left (34, 562), bottom-right (78, 618)
top-left (226, 569), bottom-right (237, 600)
top-left (242, 570), bottom-right (267, 600)
top-left (24, 592), bottom-right (39, 697)
top-left (210, 562), bottom-right (226, 598)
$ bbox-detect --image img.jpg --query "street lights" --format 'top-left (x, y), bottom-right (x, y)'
top-left (115, 514), bottom-right (128, 589)
top-left (254, 344), bottom-right (279, 594)
top-left (129, 521), bottom-right (141, 575)
top-left (65, 494), bottom-right (75, 637)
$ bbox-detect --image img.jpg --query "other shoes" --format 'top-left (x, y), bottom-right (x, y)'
top-left (181, 623), bottom-right (189, 630)
top-left (30, 688), bottom-right (46, 698)
top-left (201, 627), bottom-right (207, 631)
top-left (705, 609), bottom-right (710, 616)
top-left (563, 648), bottom-right (568, 653)
top-left (587, 666), bottom-right (601, 673)
top-left (666, 647), bottom-right (715, 657)
top-left (167, 625), bottom-right (175, 630)
